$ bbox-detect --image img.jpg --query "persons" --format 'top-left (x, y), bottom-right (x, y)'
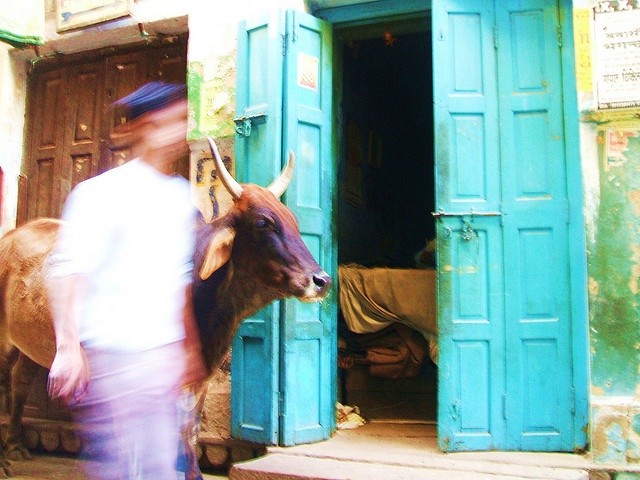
top-left (47, 80), bottom-right (208, 480)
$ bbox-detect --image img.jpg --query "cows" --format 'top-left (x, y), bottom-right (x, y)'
top-left (0, 136), bottom-right (332, 480)
